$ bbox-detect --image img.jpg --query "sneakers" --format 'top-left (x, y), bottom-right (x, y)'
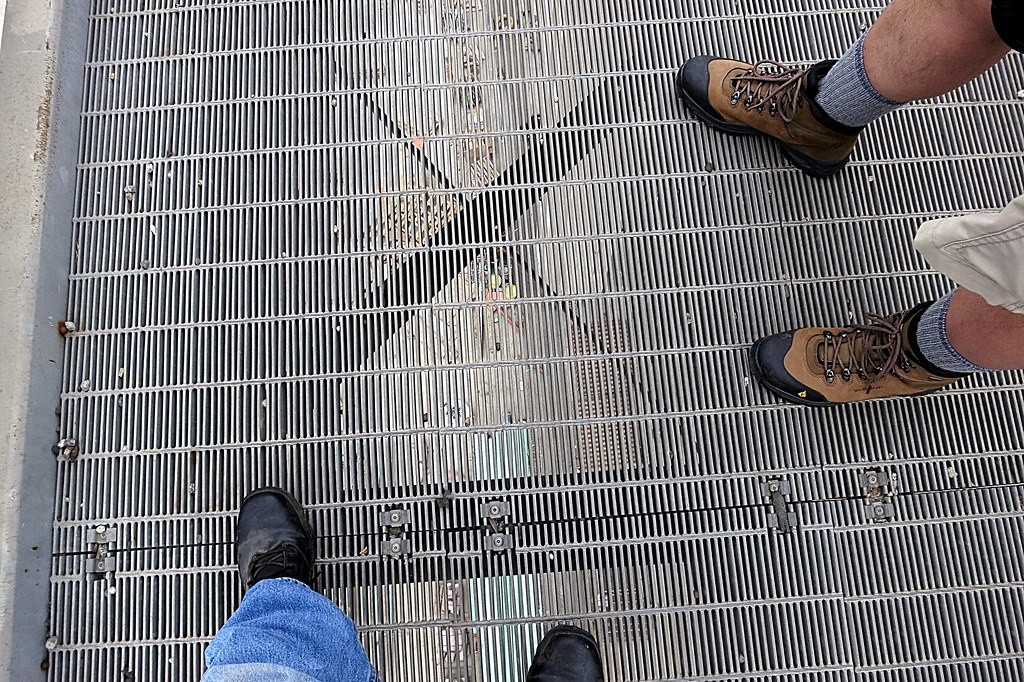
top-left (235, 487), bottom-right (313, 591)
top-left (525, 625), bottom-right (604, 682)
top-left (750, 299), bottom-right (974, 407)
top-left (676, 55), bottom-right (866, 177)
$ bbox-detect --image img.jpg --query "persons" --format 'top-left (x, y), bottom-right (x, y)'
top-left (681, 0), bottom-right (1024, 408)
top-left (200, 486), bottom-right (605, 682)
